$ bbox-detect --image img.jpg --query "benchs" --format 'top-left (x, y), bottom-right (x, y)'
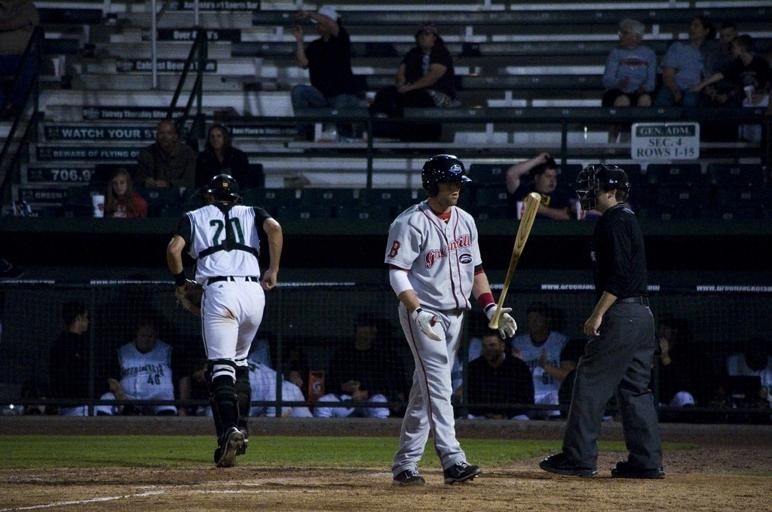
top-left (0, 0), bottom-right (771, 190)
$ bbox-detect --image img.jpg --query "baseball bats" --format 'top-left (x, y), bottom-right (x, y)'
top-left (487, 191), bottom-right (541, 329)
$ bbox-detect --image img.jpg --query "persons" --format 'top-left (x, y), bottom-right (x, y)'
top-left (104, 120), bottom-right (252, 218)
top-left (505, 151), bottom-right (585, 224)
top-left (602, 13), bottom-right (772, 144)
top-left (290, 4), bottom-right (463, 142)
top-left (313, 300), bottom-right (772, 421)
top-left (167, 173), bottom-right (282, 467)
top-left (46, 308), bottom-right (313, 418)
top-left (539, 164), bottom-right (665, 479)
top-left (1, 1), bottom-right (40, 122)
top-left (385, 155), bottom-right (517, 487)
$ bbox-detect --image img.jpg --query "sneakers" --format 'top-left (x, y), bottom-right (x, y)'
top-left (391, 470), bottom-right (424, 487)
top-left (214, 426), bottom-right (244, 467)
top-left (612, 462), bottom-right (665, 479)
top-left (444, 461), bottom-right (482, 485)
top-left (236, 426), bottom-right (248, 456)
top-left (540, 452), bottom-right (598, 477)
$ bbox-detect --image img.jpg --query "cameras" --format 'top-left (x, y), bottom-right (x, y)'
top-left (301, 17), bottom-right (320, 30)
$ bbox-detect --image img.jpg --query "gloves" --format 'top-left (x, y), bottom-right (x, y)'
top-left (483, 302), bottom-right (518, 340)
top-left (411, 306), bottom-right (442, 341)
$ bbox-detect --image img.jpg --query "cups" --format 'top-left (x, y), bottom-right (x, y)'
top-left (92, 194), bottom-right (105, 217)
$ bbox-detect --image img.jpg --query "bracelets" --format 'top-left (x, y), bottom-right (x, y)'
top-left (173, 269), bottom-right (187, 286)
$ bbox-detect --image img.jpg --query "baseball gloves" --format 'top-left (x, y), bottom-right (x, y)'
top-left (175, 280), bottom-right (203, 316)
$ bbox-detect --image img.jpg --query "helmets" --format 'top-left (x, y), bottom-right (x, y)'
top-left (575, 163), bottom-right (632, 211)
top-left (422, 153), bottom-right (473, 198)
top-left (207, 173), bottom-right (240, 199)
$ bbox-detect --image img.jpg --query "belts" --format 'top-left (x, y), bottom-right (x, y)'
top-left (617, 296), bottom-right (649, 305)
top-left (207, 276), bottom-right (257, 286)
